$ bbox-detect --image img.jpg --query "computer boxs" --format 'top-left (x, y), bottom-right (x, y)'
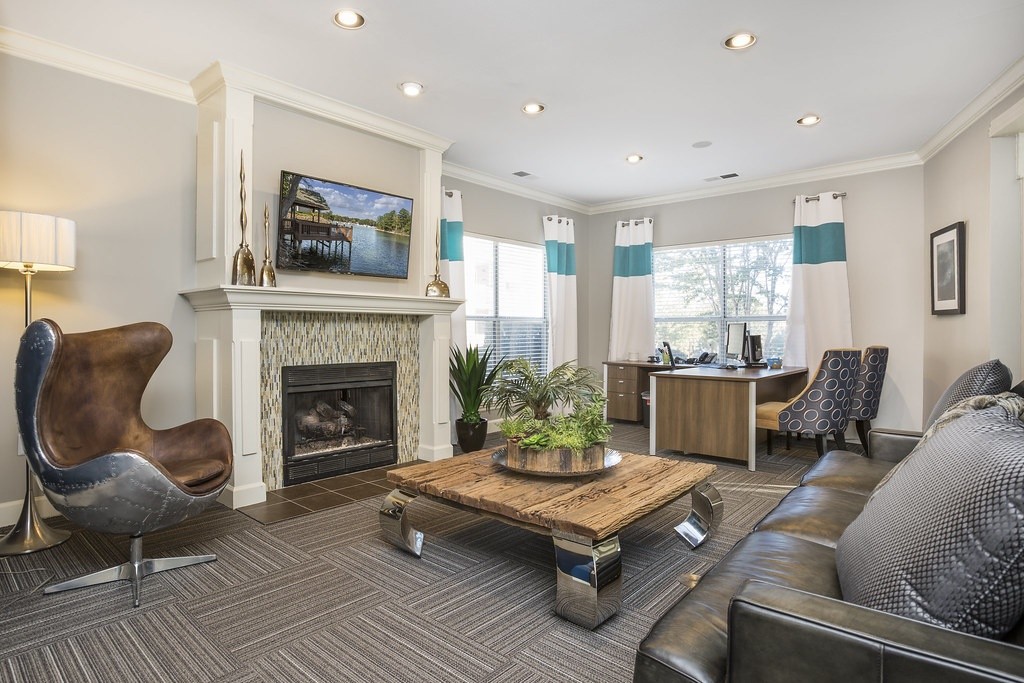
top-left (743, 335), bottom-right (762, 361)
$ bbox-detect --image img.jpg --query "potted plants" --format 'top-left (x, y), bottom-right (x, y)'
top-left (449, 341), bottom-right (508, 454)
top-left (476, 355), bottom-right (615, 472)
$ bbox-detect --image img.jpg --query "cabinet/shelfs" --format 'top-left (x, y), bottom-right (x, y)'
top-left (602, 359), bottom-right (720, 426)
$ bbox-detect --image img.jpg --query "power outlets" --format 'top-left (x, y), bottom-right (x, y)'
top-left (18, 433), bottom-right (25, 456)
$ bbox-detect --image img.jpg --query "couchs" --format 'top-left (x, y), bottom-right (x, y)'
top-left (633, 379), bottom-right (1024, 683)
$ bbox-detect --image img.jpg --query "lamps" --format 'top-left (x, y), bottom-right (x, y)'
top-left (0, 209), bottom-right (77, 555)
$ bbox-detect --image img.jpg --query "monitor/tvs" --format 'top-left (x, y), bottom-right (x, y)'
top-left (725, 323), bottom-right (749, 368)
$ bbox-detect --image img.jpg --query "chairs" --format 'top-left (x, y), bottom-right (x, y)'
top-left (0, 317), bottom-right (234, 609)
top-left (786, 344), bottom-right (889, 459)
top-left (756, 347), bottom-right (862, 458)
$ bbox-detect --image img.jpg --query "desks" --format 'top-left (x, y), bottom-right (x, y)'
top-left (647, 365), bottom-right (810, 472)
top-left (378, 445), bottom-right (725, 630)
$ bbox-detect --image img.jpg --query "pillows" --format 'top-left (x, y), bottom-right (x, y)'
top-left (835, 404), bottom-right (1024, 640)
top-left (922, 358), bottom-right (1013, 436)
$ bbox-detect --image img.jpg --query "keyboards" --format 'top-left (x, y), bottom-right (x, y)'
top-left (698, 363), bottom-right (727, 369)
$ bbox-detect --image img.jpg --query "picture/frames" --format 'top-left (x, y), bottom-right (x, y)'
top-left (930, 221), bottom-right (966, 315)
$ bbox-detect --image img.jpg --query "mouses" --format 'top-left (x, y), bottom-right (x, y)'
top-left (726, 365), bottom-right (737, 369)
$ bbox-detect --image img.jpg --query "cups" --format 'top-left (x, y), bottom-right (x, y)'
top-left (629, 351), bottom-right (639, 361)
top-left (663, 352), bottom-right (669, 364)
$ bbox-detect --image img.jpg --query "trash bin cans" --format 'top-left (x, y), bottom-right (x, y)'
top-left (641, 392), bottom-right (651, 426)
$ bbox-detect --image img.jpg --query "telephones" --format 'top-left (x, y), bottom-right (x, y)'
top-left (699, 352), bottom-right (716, 364)
